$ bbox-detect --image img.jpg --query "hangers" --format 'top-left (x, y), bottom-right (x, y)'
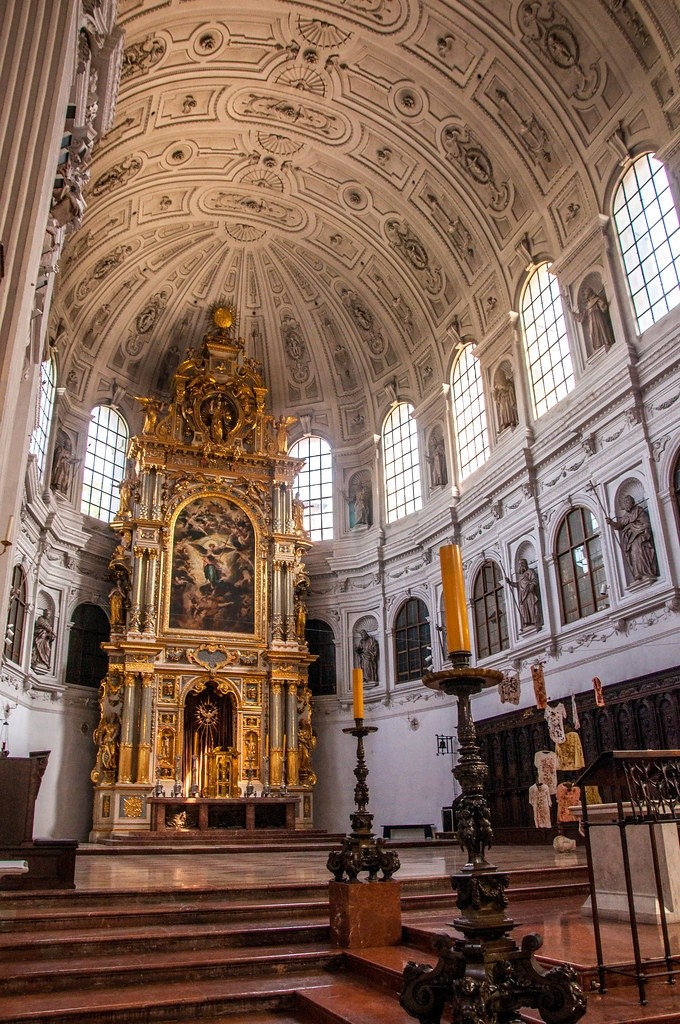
top-left (532, 658), bottom-right (576, 787)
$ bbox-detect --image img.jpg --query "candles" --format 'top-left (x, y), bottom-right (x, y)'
top-left (5, 514), bottom-right (15, 542)
top-left (440, 542), bottom-right (471, 652)
top-left (194, 731), bottom-right (198, 755)
top-left (353, 668), bottom-right (364, 719)
top-left (283, 734), bottom-right (286, 757)
top-left (265, 734), bottom-right (269, 757)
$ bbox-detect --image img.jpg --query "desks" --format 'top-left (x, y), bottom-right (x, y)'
top-left (148, 797), bottom-right (301, 831)
top-left (568, 800), bottom-right (680, 926)
